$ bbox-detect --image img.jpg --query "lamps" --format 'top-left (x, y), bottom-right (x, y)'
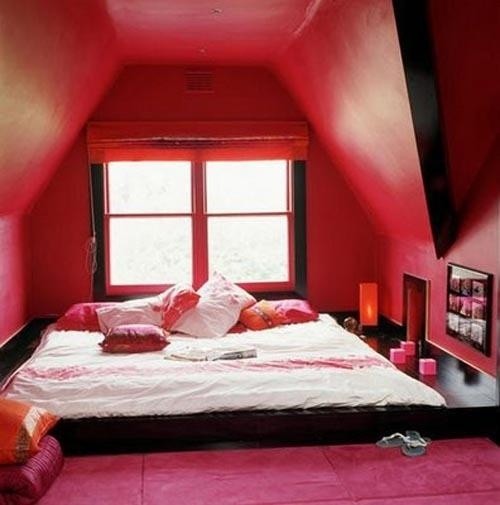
top-left (358, 282), bottom-right (379, 338)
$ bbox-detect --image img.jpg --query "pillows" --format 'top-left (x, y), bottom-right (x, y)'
top-left (53, 270), bottom-right (319, 354)
top-left (1, 400), bottom-right (61, 467)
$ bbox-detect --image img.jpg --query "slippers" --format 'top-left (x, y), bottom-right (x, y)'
top-left (376, 428), bottom-right (429, 457)
